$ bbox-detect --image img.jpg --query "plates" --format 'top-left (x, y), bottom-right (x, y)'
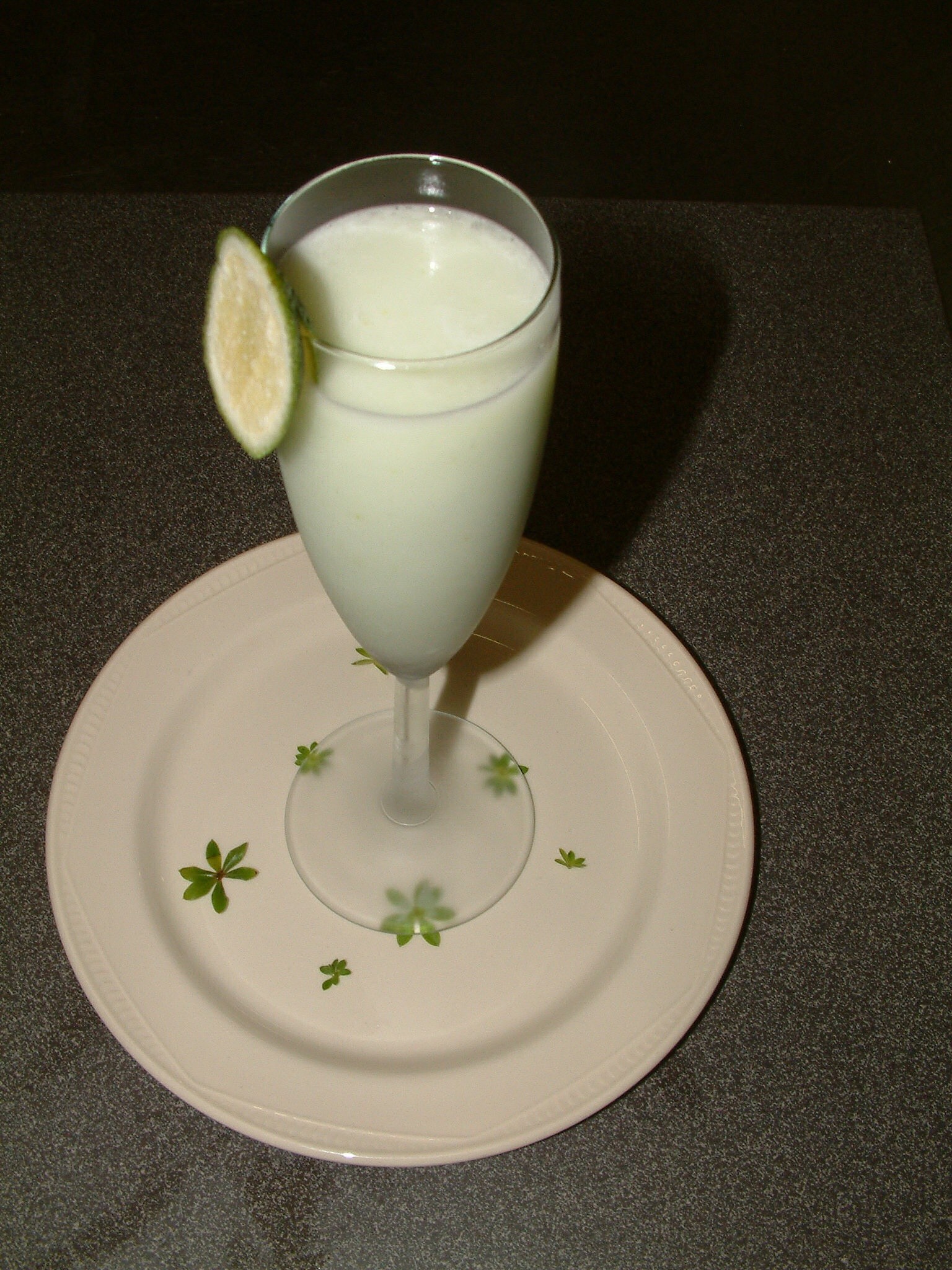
top-left (44, 529), bottom-right (752, 1167)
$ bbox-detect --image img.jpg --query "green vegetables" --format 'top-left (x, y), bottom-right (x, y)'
top-left (178, 646), bottom-right (587, 992)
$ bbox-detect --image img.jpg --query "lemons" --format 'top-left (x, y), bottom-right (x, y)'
top-left (201, 226), bottom-right (305, 459)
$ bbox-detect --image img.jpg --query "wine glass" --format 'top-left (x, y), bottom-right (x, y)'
top-left (257, 152), bottom-right (561, 935)
